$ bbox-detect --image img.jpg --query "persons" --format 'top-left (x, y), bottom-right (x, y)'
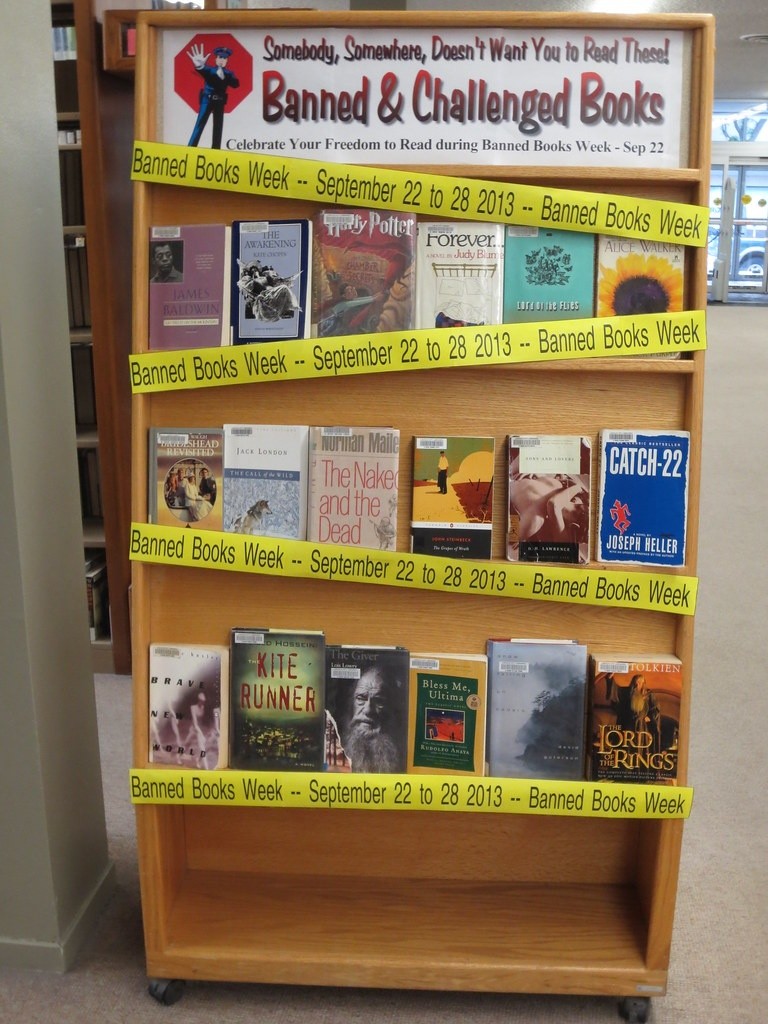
top-left (605, 671), bottom-right (660, 773)
top-left (150, 243), bottom-right (183, 283)
top-left (345, 667), bottom-right (405, 773)
top-left (166, 465), bottom-right (216, 521)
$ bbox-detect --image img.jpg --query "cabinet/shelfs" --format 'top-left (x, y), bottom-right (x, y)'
top-left (131, 6), bottom-right (718, 996)
top-left (52, 1), bottom-right (129, 684)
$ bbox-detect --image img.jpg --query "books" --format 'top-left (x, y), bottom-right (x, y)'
top-left (50, 12), bottom-right (112, 645)
top-left (147, 641), bottom-right (230, 771)
top-left (147, 206), bottom-right (687, 361)
top-left (485, 638), bottom-right (588, 782)
top-left (323, 645), bottom-right (410, 774)
top-left (406, 653), bottom-right (488, 777)
top-left (229, 626), bottom-right (325, 772)
top-left (149, 427), bottom-right (223, 532)
top-left (224, 424), bottom-right (691, 569)
top-left (589, 652), bottom-right (683, 786)
